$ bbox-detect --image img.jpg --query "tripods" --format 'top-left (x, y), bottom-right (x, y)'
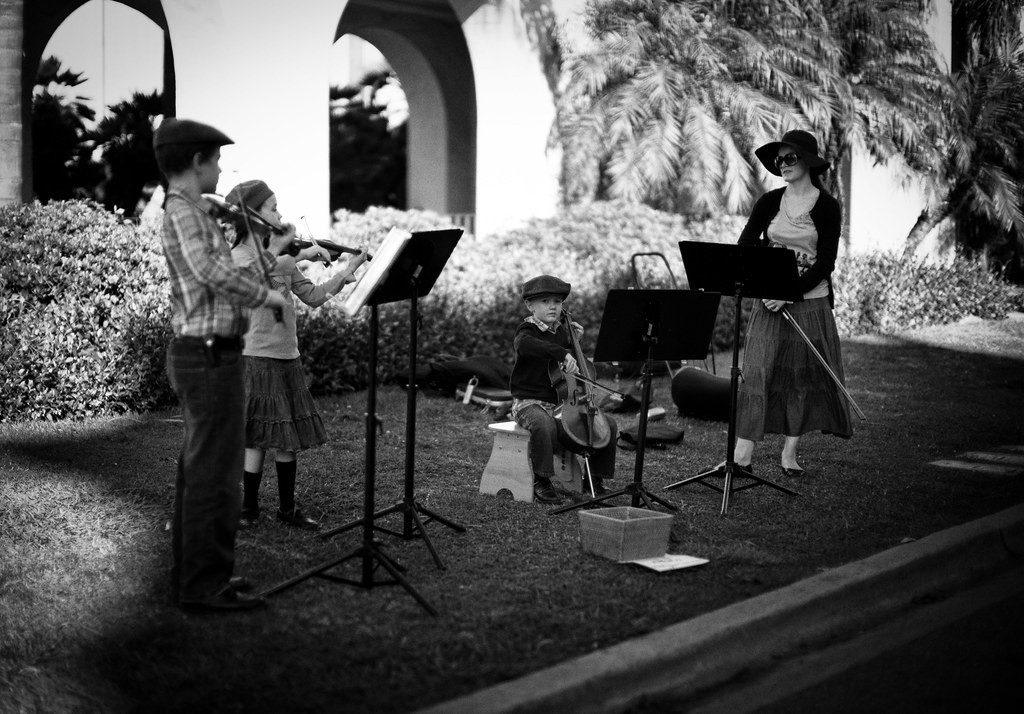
top-left (242, 223), bottom-right (466, 618)
top-left (548, 290), bottom-right (719, 514)
top-left (660, 237), bottom-right (808, 512)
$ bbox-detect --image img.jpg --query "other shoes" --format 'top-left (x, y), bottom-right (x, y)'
top-left (782, 465), bottom-right (806, 478)
top-left (169, 576), bottom-right (269, 611)
top-left (712, 463), bottom-right (753, 477)
top-left (276, 507), bottom-right (319, 530)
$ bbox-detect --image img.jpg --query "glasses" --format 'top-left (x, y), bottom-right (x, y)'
top-left (774, 152), bottom-right (799, 169)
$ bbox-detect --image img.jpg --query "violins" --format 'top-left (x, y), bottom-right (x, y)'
top-left (203, 193), bottom-right (302, 257)
top-left (292, 234), bottom-right (373, 263)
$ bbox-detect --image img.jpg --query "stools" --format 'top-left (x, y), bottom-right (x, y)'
top-left (477, 419), bottom-right (589, 505)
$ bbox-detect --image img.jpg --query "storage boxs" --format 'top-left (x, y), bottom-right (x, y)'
top-left (576, 505), bottom-right (675, 562)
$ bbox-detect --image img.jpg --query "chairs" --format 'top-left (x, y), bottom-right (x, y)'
top-left (625, 250), bottom-right (713, 392)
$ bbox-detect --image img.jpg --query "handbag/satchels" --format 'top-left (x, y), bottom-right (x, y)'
top-left (615, 420), bottom-right (684, 451)
top-left (598, 384), bottom-right (656, 413)
top-left (398, 351), bottom-right (515, 400)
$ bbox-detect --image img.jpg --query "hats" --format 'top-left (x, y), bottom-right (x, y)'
top-left (521, 274), bottom-right (571, 300)
top-left (153, 118), bottom-right (235, 146)
top-left (225, 180), bottom-right (274, 212)
top-left (756, 130), bottom-right (831, 176)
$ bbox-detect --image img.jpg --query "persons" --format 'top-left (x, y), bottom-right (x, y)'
top-left (222, 177), bottom-right (369, 531)
top-left (153, 118), bottom-right (289, 619)
top-left (691, 128), bottom-right (854, 477)
top-left (508, 273), bottom-right (618, 503)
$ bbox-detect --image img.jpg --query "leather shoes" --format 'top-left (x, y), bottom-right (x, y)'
top-left (534, 478), bottom-right (562, 504)
top-left (582, 480), bottom-right (613, 495)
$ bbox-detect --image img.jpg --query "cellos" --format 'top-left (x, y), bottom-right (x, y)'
top-left (547, 308), bottom-right (611, 499)
